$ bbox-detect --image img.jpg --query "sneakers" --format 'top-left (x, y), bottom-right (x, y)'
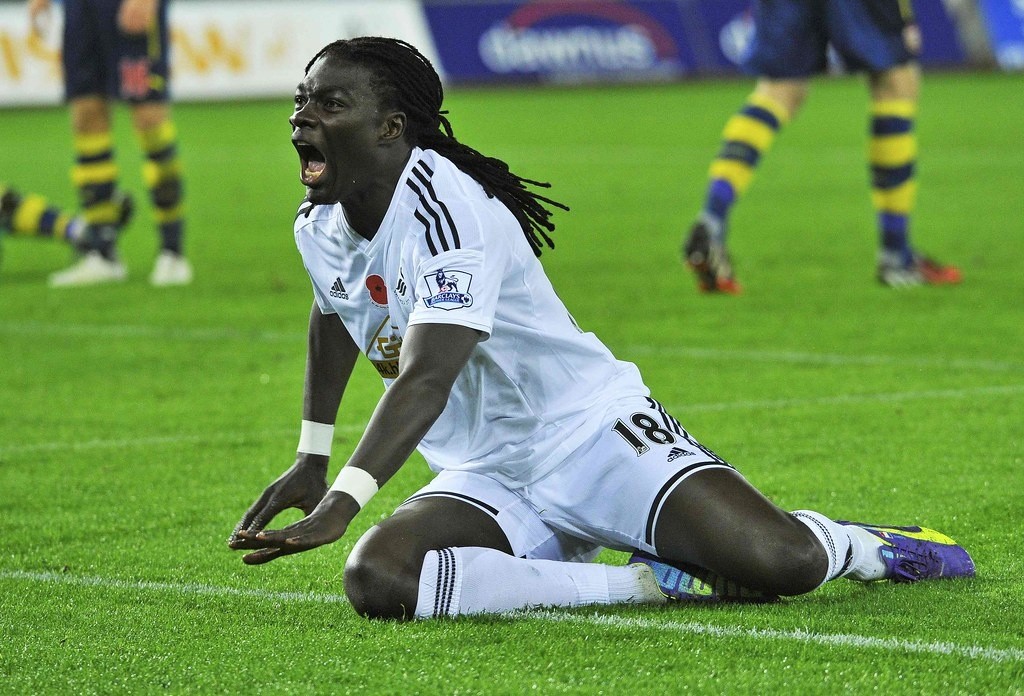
top-left (684, 219), bottom-right (740, 294)
top-left (878, 254), bottom-right (963, 288)
top-left (836, 520), bottom-right (976, 583)
top-left (628, 549), bottom-right (781, 603)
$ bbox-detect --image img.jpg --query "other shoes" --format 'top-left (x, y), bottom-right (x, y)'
top-left (75, 195), bottom-right (133, 251)
top-left (48, 249), bottom-right (125, 286)
top-left (151, 250), bottom-right (192, 285)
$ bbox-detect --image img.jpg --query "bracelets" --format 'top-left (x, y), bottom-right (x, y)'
top-left (328, 466), bottom-right (379, 511)
top-left (297, 420), bottom-right (334, 456)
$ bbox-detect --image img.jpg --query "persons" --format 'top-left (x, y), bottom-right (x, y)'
top-left (0, 183), bottom-right (136, 258)
top-left (227, 37), bottom-right (975, 626)
top-left (47, 0), bottom-right (193, 291)
top-left (682, 1), bottom-right (961, 292)
